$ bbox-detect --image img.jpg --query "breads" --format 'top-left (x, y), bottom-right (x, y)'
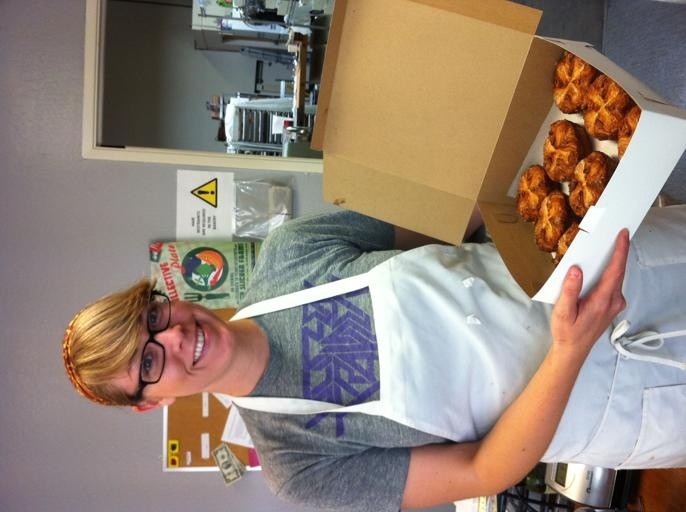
top-left (534, 190), bottom-right (576, 252)
top-left (552, 50), bottom-right (597, 115)
top-left (568, 150), bottom-right (616, 219)
top-left (542, 118), bottom-right (593, 183)
top-left (551, 220), bottom-right (579, 268)
top-left (618, 104), bottom-right (641, 160)
top-left (514, 163), bottom-right (562, 224)
top-left (583, 72), bottom-right (632, 140)
